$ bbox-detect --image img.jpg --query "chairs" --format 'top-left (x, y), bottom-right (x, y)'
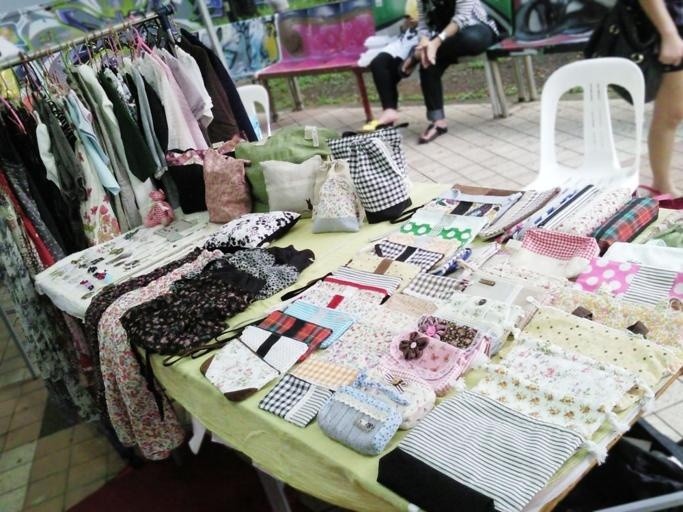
top-left (519, 57), bottom-right (645, 196)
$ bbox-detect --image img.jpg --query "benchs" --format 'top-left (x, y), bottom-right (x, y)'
top-left (190, 1), bottom-right (616, 123)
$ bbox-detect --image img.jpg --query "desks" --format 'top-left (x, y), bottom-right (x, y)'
top-left (34, 181), bottom-right (683, 512)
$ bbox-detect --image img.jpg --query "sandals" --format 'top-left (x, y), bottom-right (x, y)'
top-left (398, 44), bottom-right (420, 78)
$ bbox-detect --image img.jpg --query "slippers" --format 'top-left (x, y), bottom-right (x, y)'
top-left (418, 122), bottom-right (449, 145)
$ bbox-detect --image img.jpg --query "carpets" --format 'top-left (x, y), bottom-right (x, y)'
top-left (66, 431), bottom-right (315, 512)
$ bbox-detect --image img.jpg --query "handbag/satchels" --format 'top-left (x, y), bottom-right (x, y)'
top-left (324, 118), bottom-right (414, 226)
top-left (311, 157), bottom-right (366, 235)
top-left (165, 133), bottom-right (245, 214)
top-left (234, 122), bottom-right (343, 213)
top-left (580, 6), bottom-right (664, 106)
top-left (201, 147), bottom-right (252, 225)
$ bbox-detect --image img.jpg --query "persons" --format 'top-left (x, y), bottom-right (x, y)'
top-left (637, 1), bottom-right (683, 200)
top-left (412, 0), bottom-right (507, 144)
top-left (358, 0), bottom-right (432, 132)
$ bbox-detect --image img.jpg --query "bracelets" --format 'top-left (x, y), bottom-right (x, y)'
top-left (439, 32), bottom-right (447, 44)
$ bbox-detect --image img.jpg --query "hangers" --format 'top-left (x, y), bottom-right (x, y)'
top-left (0, 2), bottom-right (214, 147)
